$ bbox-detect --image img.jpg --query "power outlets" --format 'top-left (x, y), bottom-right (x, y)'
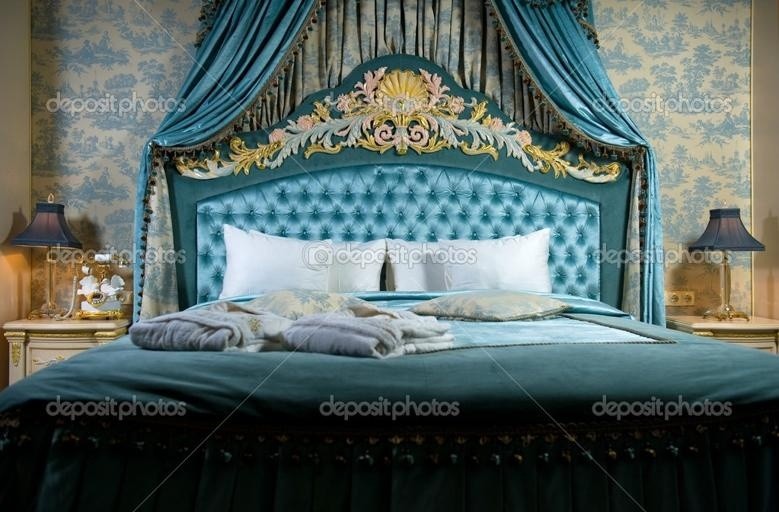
top-left (664, 290), bottom-right (695, 307)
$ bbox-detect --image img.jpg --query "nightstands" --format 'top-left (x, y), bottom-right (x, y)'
top-left (1, 318), bottom-right (133, 389)
top-left (665, 315), bottom-right (779, 354)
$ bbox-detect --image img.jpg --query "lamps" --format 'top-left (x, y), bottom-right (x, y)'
top-left (688, 208), bottom-right (766, 322)
top-left (10, 192), bottom-right (83, 320)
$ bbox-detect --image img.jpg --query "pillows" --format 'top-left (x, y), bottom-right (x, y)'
top-left (215, 222), bottom-right (572, 322)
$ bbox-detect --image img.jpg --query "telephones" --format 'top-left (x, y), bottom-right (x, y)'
top-left (79, 254), bottom-right (131, 319)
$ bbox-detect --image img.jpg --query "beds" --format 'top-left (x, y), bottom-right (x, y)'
top-left (0, 51), bottom-right (779, 512)
